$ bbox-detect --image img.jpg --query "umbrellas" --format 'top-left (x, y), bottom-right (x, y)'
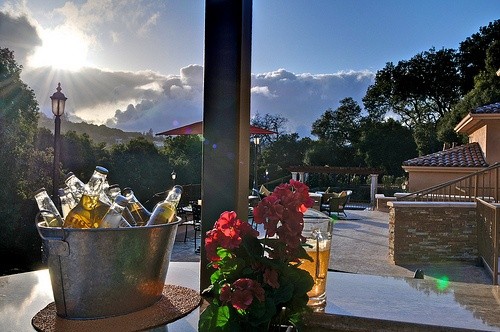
top-left (155, 120), bottom-right (278, 136)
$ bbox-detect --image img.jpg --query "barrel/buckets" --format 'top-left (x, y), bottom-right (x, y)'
top-left (34, 209), bottom-right (181, 321)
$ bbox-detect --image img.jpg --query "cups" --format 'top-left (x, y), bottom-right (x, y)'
top-left (289, 216), bottom-right (333, 306)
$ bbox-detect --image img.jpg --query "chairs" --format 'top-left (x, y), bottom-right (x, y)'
top-left (174, 204), bottom-right (202, 252)
top-left (322, 190), bottom-right (353, 217)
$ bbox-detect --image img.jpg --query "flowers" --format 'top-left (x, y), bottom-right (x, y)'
top-left (197, 177), bottom-right (315, 332)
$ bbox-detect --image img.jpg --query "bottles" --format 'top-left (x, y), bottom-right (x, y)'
top-left (34, 165), bottom-right (183, 228)
